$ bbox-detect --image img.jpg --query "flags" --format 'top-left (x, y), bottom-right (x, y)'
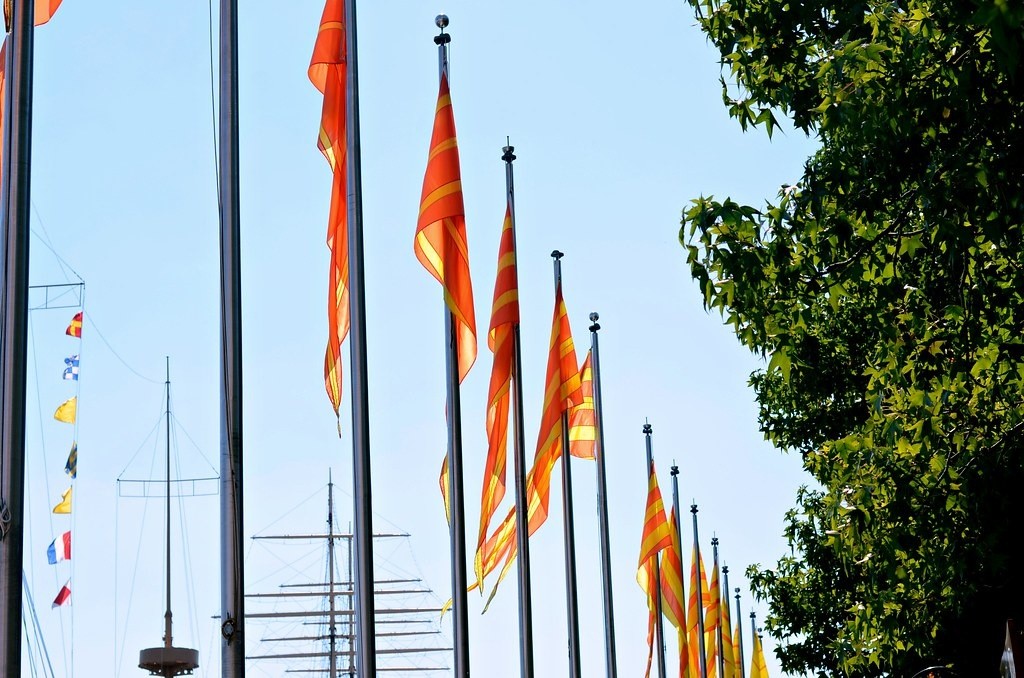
top-left (63, 442), bottom-right (78, 481)
top-left (66, 312), bottom-right (83, 338)
top-left (50, 575), bottom-right (73, 609)
top-left (52, 485), bottom-right (73, 515)
top-left (53, 393), bottom-right (77, 425)
top-left (62, 353), bottom-right (80, 383)
top-left (46, 528), bottom-right (73, 565)
